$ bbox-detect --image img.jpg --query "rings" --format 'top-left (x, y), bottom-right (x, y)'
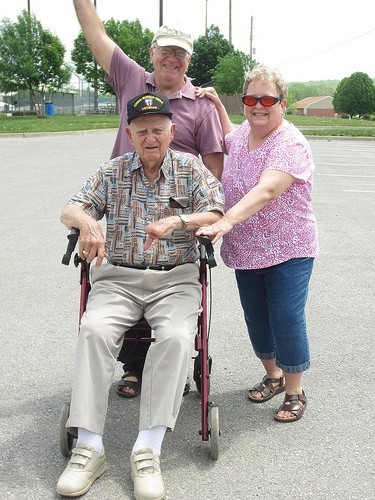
top-left (83, 250), bottom-right (89, 256)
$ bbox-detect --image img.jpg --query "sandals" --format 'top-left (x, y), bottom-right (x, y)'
top-left (248, 375), bottom-right (285, 402)
top-left (117, 365), bottom-right (142, 397)
top-left (274, 389), bottom-right (307, 421)
top-left (183, 377), bottom-right (190, 396)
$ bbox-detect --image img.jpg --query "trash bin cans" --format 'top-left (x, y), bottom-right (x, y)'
top-left (44, 102), bottom-right (55, 116)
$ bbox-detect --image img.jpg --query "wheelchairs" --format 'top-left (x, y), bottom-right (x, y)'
top-left (60, 227), bottom-right (222, 459)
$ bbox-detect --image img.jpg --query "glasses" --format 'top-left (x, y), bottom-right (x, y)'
top-left (241, 95), bottom-right (282, 107)
top-left (154, 47), bottom-right (187, 57)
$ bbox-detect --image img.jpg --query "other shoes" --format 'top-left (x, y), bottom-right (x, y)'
top-left (129, 448), bottom-right (165, 500)
top-left (57, 443), bottom-right (106, 496)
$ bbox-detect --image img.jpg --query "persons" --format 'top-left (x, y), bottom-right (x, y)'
top-left (55, 92), bottom-right (226, 500)
top-left (72, 0), bottom-right (224, 399)
top-left (194, 64), bottom-right (317, 422)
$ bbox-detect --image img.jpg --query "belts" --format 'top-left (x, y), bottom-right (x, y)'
top-left (113, 262), bottom-right (195, 271)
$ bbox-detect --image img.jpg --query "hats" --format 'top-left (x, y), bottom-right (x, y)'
top-left (152, 25), bottom-right (193, 55)
top-left (127, 92), bottom-right (172, 124)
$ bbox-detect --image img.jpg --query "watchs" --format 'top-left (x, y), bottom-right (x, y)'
top-left (179, 214), bottom-right (191, 230)
